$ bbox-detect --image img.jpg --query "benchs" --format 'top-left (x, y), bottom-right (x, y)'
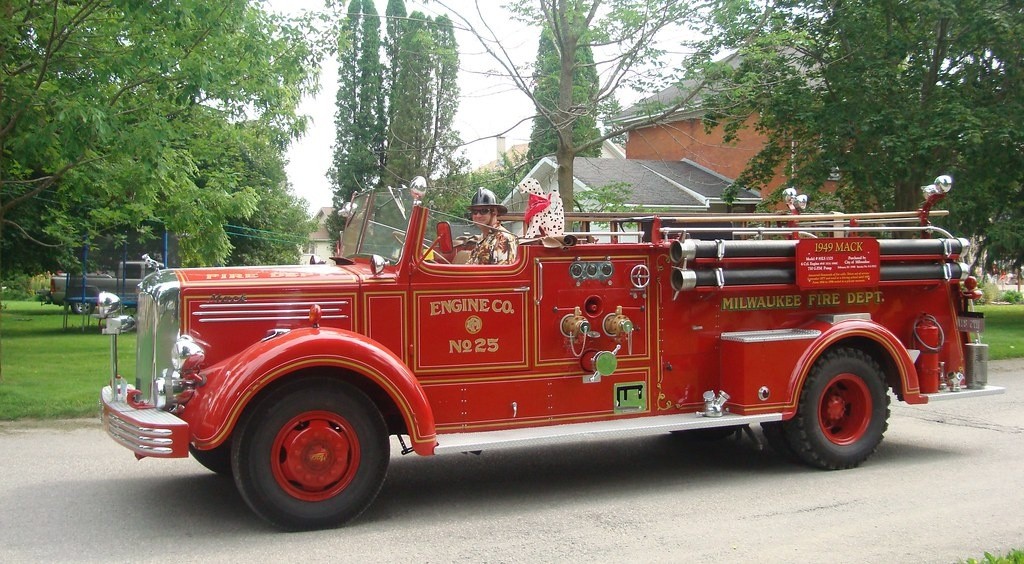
top-left (451, 250), bottom-right (472, 264)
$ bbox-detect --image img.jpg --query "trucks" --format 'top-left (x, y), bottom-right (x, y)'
top-left (98, 164), bottom-right (989, 532)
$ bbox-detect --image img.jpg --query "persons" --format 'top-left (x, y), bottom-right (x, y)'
top-left (464, 186), bottom-right (518, 265)
top-left (996, 259), bottom-right (1024, 285)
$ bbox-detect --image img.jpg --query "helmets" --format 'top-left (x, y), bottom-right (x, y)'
top-left (467, 187), bottom-right (508, 217)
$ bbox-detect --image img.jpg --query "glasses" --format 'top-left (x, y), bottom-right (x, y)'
top-left (470, 209), bottom-right (491, 215)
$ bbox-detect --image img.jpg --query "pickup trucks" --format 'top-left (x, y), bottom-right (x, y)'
top-left (44, 261), bottom-right (169, 314)
